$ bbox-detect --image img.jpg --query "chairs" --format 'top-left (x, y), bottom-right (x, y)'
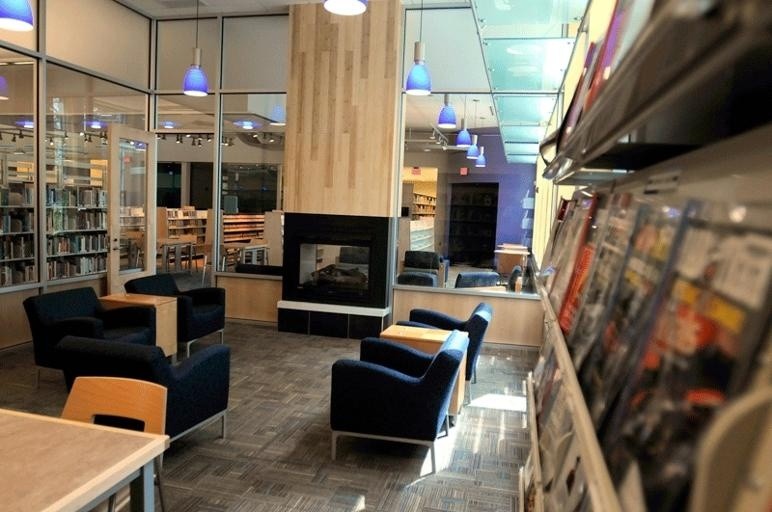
top-left (60, 376), bottom-right (168, 432)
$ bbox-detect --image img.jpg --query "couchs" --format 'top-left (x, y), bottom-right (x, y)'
top-left (58, 328), bottom-right (235, 475)
top-left (455, 271), bottom-right (499, 287)
top-left (122, 269), bottom-right (231, 361)
top-left (391, 267), bottom-right (438, 287)
top-left (394, 298), bottom-right (494, 387)
top-left (324, 329), bottom-right (472, 473)
top-left (20, 284), bottom-right (160, 373)
top-left (401, 249), bottom-right (450, 289)
top-left (508, 266), bottom-right (524, 291)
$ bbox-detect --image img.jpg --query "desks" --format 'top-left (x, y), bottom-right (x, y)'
top-left (221, 242), bottom-right (268, 271)
top-left (160, 241), bottom-right (201, 270)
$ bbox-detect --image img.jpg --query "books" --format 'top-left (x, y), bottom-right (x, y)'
top-left (45, 183), bottom-right (106, 207)
top-left (44, 208), bottom-right (105, 231)
top-left (47, 257), bottom-right (105, 278)
top-left (0, 209), bottom-right (33, 232)
top-left (0, 235), bottom-right (34, 259)
top-left (1, 261), bottom-right (36, 287)
top-left (520, 187), bottom-right (772, 512)
top-left (44, 234), bottom-right (109, 256)
top-left (0, 178), bottom-right (36, 206)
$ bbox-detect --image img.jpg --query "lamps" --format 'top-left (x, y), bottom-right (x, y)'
top-left (180, 3), bottom-right (208, 96)
top-left (0, 0), bottom-right (34, 32)
top-left (436, 94), bottom-right (458, 131)
top-left (455, 95), bottom-right (471, 148)
top-left (401, 0), bottom-right (434, 95)
top-left (467, 97), bottom-right (479, 160)
top-left (475, 114), bottom-right (486, 169)
top-left (323, 0), bottom-right (368, 17)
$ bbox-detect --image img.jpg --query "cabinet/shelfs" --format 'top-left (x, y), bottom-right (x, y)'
top-left (1, 182), bottom-right (108, 286)
top-left (157, 209), bottom-right (210, 240)
top-left (211, 213), bottom-right (265, 244)
